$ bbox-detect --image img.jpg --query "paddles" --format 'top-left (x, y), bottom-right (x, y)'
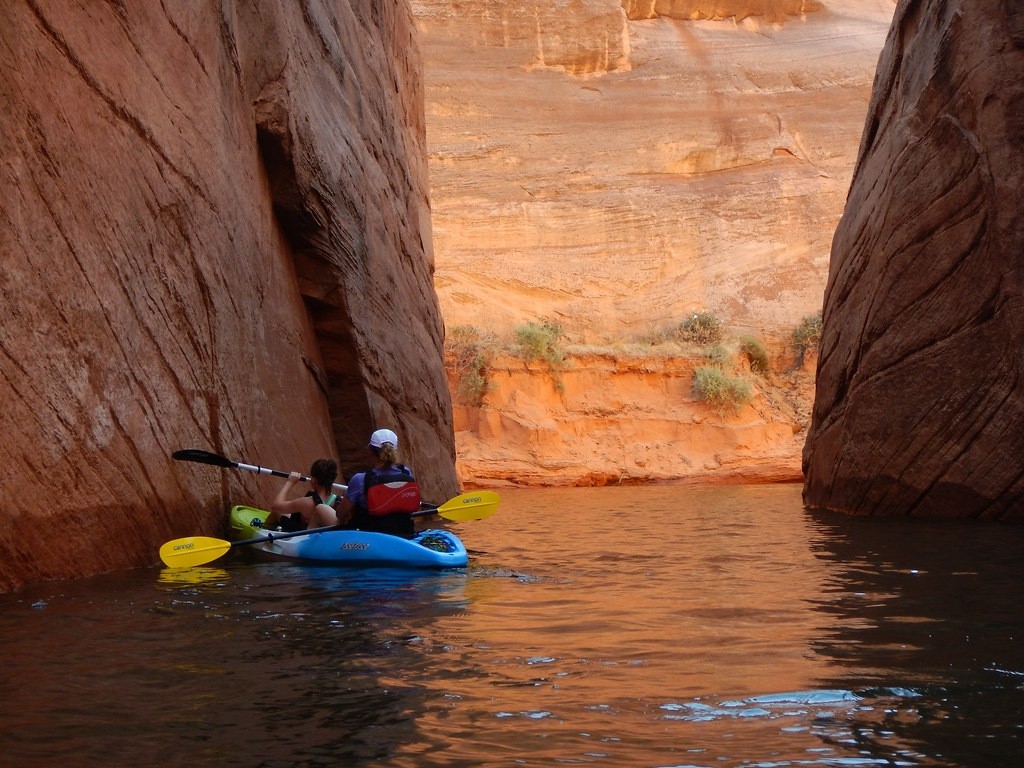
top-left (173, 449), bottom-right (440, 510)
top-left (159, 491), bottom-right (498, 569)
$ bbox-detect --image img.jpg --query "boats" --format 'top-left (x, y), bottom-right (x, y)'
top-left (230, 504), bottom-right (469, 568)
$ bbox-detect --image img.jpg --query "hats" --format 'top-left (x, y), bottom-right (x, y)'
top-left (370, 429), bottom-right (398, 449)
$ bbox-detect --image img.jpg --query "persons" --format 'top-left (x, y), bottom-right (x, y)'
top-left (262, 457), bottom-right (343, 535)
top-left (306, 428), bottom-right (417, 539)
